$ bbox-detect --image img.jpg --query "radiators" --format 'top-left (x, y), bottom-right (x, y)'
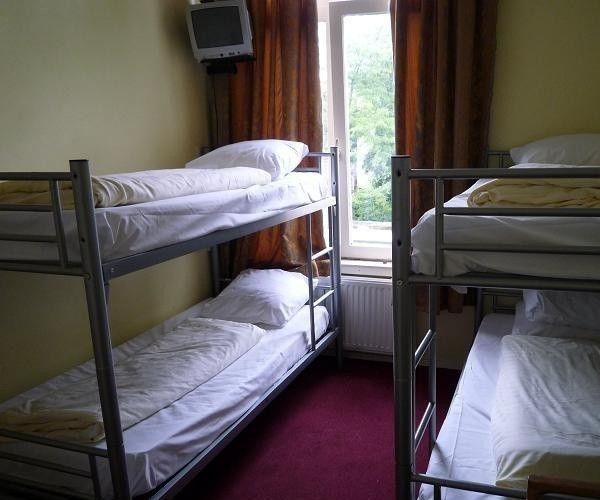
top-left (304, 275), bottom-right (393, 356)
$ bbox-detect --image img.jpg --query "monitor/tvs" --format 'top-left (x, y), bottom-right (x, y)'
top-left (186, 0), bottom-right (254, 62)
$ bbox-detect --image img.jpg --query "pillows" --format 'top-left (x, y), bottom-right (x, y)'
top-left (521, 289), bottom-right (600, 329)
top-left (197, 267), bottom-right (319, 329)
top-left (183, 138), bottom-right (309, 181)
top-left (511, 301), bottom-right (600, 337)
top-left (509, 133), bottom-right (600, 165)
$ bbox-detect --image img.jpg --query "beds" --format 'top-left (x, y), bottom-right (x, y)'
top-left (0, 146), bottom-right (344, 500)
top-left (390, 146), bottom-right (600, 500)
